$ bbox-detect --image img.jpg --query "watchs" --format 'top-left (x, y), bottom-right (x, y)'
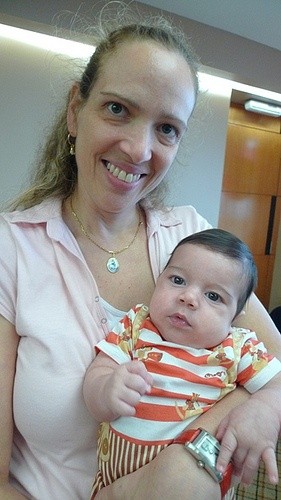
top-left (171, 427), bottom-right (233, 498)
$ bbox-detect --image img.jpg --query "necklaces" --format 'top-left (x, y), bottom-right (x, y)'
top-left (68, 193), bottom-right (142, 272)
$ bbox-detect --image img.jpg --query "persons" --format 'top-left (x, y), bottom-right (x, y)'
top-left (1, 26), bottom-right (281, 500)
top-left (83, 229), bottom-right (281, 500)
